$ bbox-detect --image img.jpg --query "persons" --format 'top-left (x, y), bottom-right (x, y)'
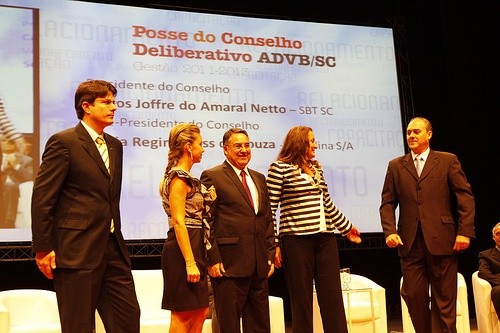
top-left (205, 274), bottom-right (221, 333)
top-left (0, 98), bottom-right (30, 228)
top-left (199, 128), bottom-right (276, 333)
top-left (380, 117), bottom-right (477, 333)
top-left (266, 126), bottom-right (362, 333)
top-left (160, 123), bottom-right (217, 333)
top-left (478, 223), bottom-right (500, 319)
top-left (31, 80), bottom-right (141, 333)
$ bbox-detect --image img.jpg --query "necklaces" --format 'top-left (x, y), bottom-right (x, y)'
top-left (293, 162), bottom-right (320, 187)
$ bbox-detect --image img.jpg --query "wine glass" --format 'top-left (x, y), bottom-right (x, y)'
top-left (341, 267), bottom-right (351, 291)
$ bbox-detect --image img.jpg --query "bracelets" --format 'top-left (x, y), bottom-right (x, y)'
top-left (186, 262), bottom-right (196, 267)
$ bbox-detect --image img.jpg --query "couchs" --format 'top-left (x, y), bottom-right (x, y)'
top-left (399, 271), bottom-right (471, 333)
top-left (95, 269), bottom-right (285, 333)
top-left (312, 273), bottom-right (388, 333)
top-left (471, 270), bottom-right (500, 333)
top-left (0, 289), bottom-right (62, 333)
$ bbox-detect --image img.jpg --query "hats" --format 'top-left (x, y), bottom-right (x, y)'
top-left (240, 170), bottom-right (253, 205)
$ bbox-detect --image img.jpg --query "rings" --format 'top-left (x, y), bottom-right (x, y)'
top-left (38, 267), bottom-right (42, 269)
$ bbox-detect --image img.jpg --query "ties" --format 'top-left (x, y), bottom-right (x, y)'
top-left (414, 156), bottom-right (422, 177)
top-left (95, 136), bottom-right (115, 234)
